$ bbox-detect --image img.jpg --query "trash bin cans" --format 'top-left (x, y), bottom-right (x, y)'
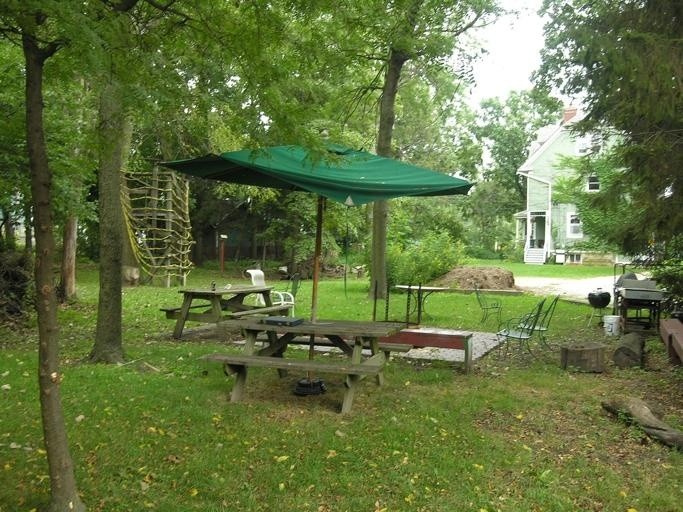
top-left (555, 249), bottom-right (565, 264)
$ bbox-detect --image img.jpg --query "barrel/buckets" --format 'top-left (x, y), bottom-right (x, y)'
top-left (602, 315), bottom-right (621, 338)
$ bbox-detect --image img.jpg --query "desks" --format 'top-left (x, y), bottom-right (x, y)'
top-left (395, 285), bottom-right (450, 320)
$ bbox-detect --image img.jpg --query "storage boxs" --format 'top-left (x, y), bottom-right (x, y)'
top-left (560, 342), bottom-right (606, 373)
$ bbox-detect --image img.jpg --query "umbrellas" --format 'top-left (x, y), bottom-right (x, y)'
top-left (156, 130), bottom-right (477, 395)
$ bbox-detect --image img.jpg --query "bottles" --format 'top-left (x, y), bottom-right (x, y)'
top-left (210, 279), bottom-right (216, 291)
top-left (224, 284), bottom-right (232, 290)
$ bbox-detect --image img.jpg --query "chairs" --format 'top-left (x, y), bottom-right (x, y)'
top-left (491, 298), bottom-right (546, 367)
top-left (517, 296), bottom-right (559, 351)
top-left (246, 269), bottom-right (294, 318)
top-left (474, 283), bottom-right (502, 325)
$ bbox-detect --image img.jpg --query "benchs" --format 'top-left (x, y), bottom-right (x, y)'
top-left (379, 332), bottom-right (473, 376)
top-left (160, 285), bottom-right (413, 416)
top-left (659, 318), bottom-right (683, 364)
